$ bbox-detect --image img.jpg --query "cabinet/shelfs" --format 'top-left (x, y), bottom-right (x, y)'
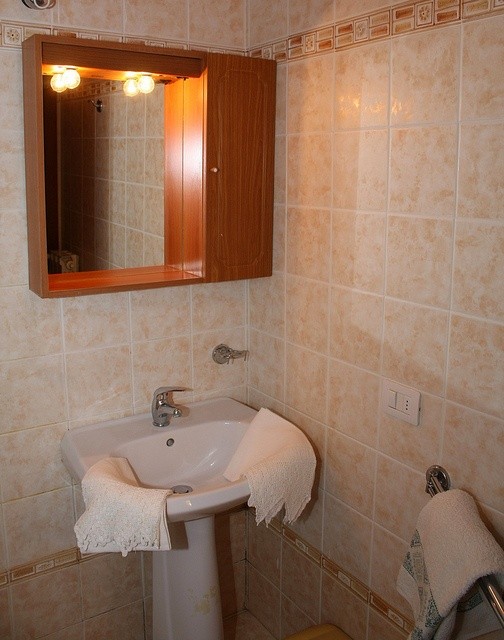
top-left (206, 52), bottom-right (275, 283)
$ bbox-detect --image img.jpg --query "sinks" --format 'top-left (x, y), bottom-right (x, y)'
top-left (82, 421), bottom-right (278, 515)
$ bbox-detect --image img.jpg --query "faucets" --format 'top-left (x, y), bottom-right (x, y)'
top-left (151, 386), bottom-right (187, 427)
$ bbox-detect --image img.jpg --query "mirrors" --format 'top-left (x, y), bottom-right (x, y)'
top-left (21, 35), bottom-right (279, 298)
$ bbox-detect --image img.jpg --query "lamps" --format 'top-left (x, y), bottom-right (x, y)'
top-left (49, 72), bottom-right (65, 94)
top-left (121, 78), bottom-right (139, 96)
top-left (66, 72), bottom-right (80, 89)
top-left (138, 75), bottom-right (156, 94)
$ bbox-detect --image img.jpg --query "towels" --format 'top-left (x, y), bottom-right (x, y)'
top-left (72, 457), bottom-right (173, 560)
top-left (396, 491), bottom-right (503, 639)
top-left (221, 406), bottom-right (318, 529)
